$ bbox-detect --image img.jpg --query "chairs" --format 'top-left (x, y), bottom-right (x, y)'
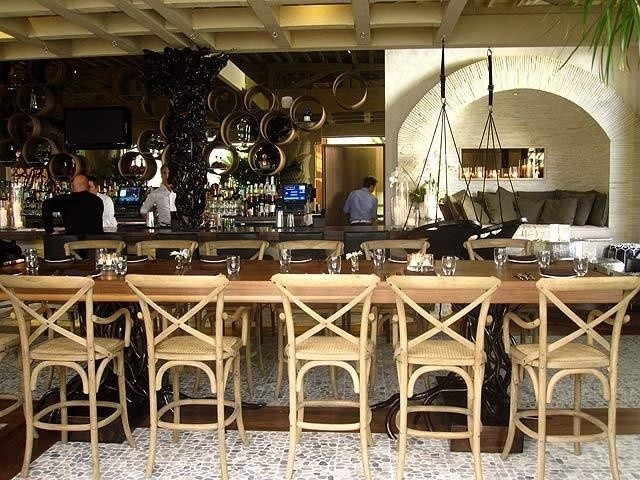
top-left (125, 274), bottom-right (251, 480)
top-left (463, 238), bottom-right (538, 386)
top-left (280, 232), bottom-right (327, 263)
top-left (271, 273), bottom-right (380, 480)
top-left (216, 232), bottom-right (274, 342)
top-left (455, 49), bottom-right (522, 260)
top-left (386, 275), bottom-right (501, 480)
top-left (86, 234), bottom-right (128, 257)
top-left (44, 235), bottom-right (78, 258)
top-left (45, 240), bottom-right (126, 389)
top-left (0, 304), bottom-right (40, 440)
top-left (274, 240), bottom-right (344, 401)
top-left (194, 240), bottom-right (270, 396)
top-left (402, 35), bottom-right (480, 259)
top-left (0, 275), bottom-right (136, 480)
top-left (134, 240), bottom-right (198, 343)
top-left (360, 240), bottom-right (430, 391)
top-left (342, 231), bottom-right (389, 343)
top-left (155, 233), bottom-right (199, 259)
top-left (500, 277), bottom-right (640, 480)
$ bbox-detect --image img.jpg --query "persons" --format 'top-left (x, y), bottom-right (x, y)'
top-left (140, 163), bottom-right (177, 230)
top-left (343, 176), bottom-right (378, 227)
top-left (88, 180), bottom-right (118, 232)
top-left (42, 174), bottom-right (104, 234)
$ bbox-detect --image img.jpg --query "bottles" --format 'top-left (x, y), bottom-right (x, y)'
top-left (97, 180), bottom-right (119, 195)
top-left (206, 174), bottom-right (284, 232)
top-left (146, 211), bottom-right (155, 229)
top-left (286, 212), bottom-right (295, 228)
top-left (30, 88), bottom-right (37, 108)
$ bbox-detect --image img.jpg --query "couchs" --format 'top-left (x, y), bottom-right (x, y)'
top-left (468, 223), bottom-right (613, 260)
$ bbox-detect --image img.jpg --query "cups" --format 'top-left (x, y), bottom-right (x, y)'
top-left (462, 159), bottom-right (532, 178)
top-left (25, 247), bottom-right (588, 280)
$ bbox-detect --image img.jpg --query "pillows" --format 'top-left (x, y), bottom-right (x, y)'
top-left (484, 192), bottom-right (517, 225)
top-left (585, 190), bottom-right (609, 227)
top-left (496, 186), bottom-right (513, 194)
top-left (517, 192), bottom-right (558, 201)
top-left (513, 199), bottom-right (545, 223)
top-left (452, 189), bottom-right (469, 204)
top-left (555, 189), bottom-right (597, 226)
top-left (477, 191), bottom-right (496, 207)
top-left (460, 193), bottom-right (489, 223)
top-left (539, 198), bottom-right (578, 225)
top-left (446, 195), bottom-right (464, 220)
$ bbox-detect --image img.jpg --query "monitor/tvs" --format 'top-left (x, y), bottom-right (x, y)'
top-left (116, 186), bottom-right (145, 206)
top-left (282, 183), bottom-right (310, 204)
top-left (64, 105), bottom-right (132, 150)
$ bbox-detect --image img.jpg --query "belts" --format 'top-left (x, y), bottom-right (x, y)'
top-left (159, 223), bottom-right (172, 227)
top-left (350, 219), bottom-right (371, 224)
